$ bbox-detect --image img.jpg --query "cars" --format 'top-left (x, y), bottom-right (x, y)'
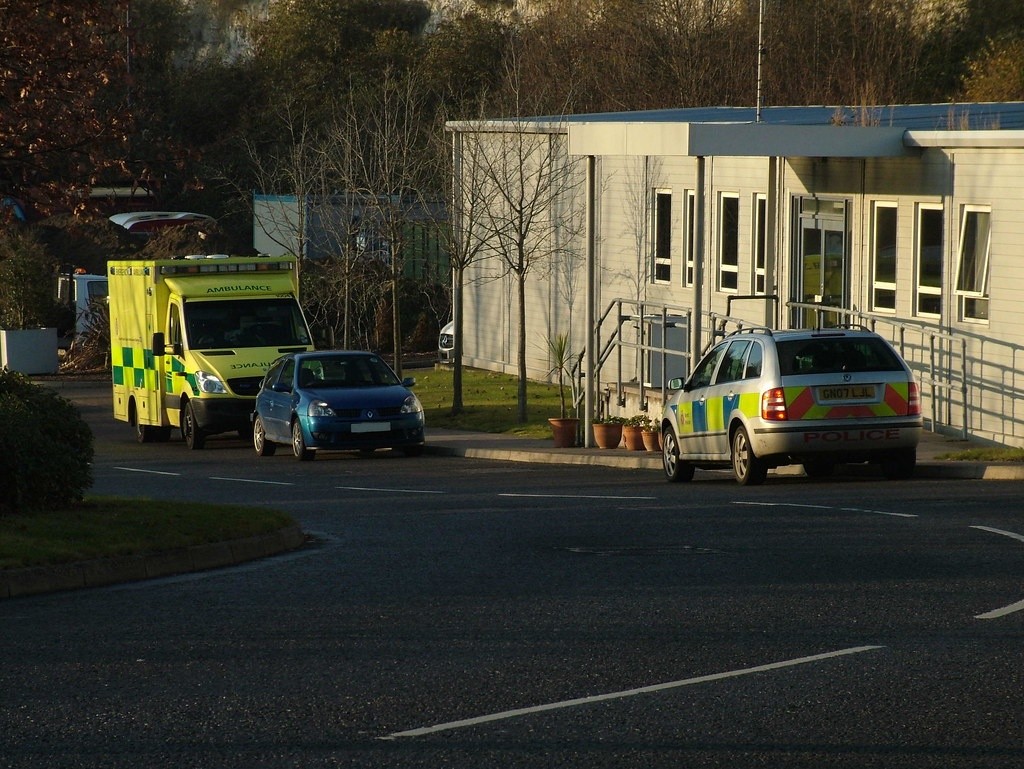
top-left (252, 349), bottom-right (426, 462)
top-left (438, 320), bottom-right (455, 365)
top-left (661, 324), bottom-right (925, 487)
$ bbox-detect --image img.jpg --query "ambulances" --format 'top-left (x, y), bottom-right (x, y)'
top-left (107, 253), bottom-right (326, 451)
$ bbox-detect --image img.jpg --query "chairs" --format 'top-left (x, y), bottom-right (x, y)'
top-left (299, 368), bottom-right (316, 387)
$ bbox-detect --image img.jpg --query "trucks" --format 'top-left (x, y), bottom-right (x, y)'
top-left (55, 267), bottom-right (110, 361)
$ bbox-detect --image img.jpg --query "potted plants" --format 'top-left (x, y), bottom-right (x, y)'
top-left (528, 330), bottom-right (581, 448)
top-left (590, 415), bottom-right (627, 449)
top-left (641, 419), bottom-right (660, 451)
top-left (622, 416), bottom-right (646, 452)
top-left (0, 225), bottom-right (58, 376)
